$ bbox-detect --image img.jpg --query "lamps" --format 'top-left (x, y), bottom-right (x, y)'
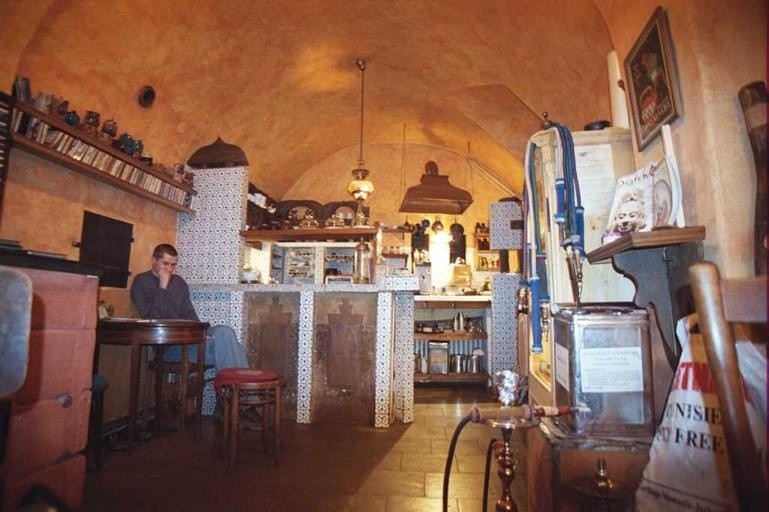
top-left (347, 58), bottom-right (374, 201)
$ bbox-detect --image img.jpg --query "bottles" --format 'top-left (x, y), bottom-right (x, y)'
top-left (85, 110), bottom-right (118, 137)
top-left (476, 222), bottom-right (491, 269)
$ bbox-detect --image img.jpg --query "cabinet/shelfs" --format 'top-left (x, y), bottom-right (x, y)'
top-left (551, 226), bottom-right (709, 443)
top-left (409, 303), bottom-right (497, 384)
top-left (259, 234), bottom-right (376, 289)
top-left (0, 89), bottom-right (199, 217)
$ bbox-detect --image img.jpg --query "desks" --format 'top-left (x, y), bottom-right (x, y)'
top-left (88, 317), bottom-right (211, 458)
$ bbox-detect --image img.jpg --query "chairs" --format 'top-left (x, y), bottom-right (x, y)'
top-left (688, 261), bottom-right (769, 511)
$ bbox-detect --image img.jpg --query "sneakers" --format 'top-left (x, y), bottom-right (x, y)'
top-left (212, 398), bottom-right (273, 431)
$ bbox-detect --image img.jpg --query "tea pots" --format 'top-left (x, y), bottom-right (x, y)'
top-left (35, 90), bottom-right (70, 122)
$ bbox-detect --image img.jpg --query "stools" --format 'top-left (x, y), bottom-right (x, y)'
top-left (215, 367), bottom-right (288, 475)
top-left (147, 359), bottom-right (217, 427)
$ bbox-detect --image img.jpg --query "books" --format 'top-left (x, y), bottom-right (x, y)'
top-left (13, 85), bottom-right (193, 208)
top-left (651, 149), bottom-right (680, 228)
top-left (607, 164), bottom-right (656, 233)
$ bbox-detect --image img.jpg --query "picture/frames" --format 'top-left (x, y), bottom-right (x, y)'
top-left (621, 7), bottom-right (684, 153)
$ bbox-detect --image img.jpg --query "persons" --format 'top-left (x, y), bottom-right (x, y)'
top-left (130, 244), bottom-right (273, 432)
top-left (609, 187), bottom-right (648, 233)
